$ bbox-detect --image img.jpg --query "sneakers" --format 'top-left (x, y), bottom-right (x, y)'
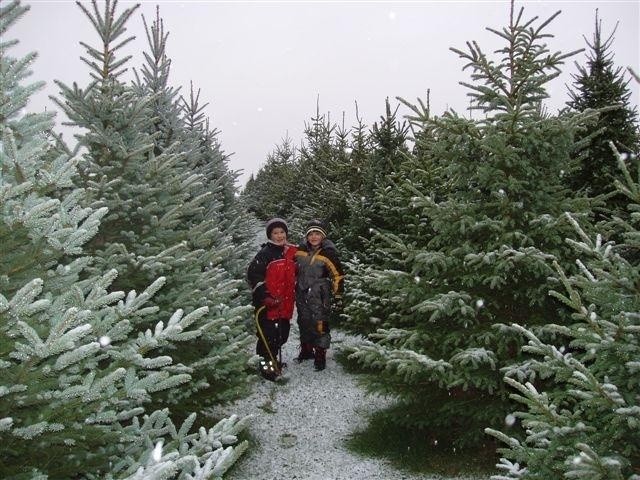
top-left (261, 370), bottom-right (286, 384)
top-left (292, 350), bottom-right (315, 362)
top-left (315, 358), bottom-right (326, 371)
top-left (277, 361), bottom-right (287, 368)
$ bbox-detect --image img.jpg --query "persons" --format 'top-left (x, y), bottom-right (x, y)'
top-left (294, 220), bottom-right (345, 372)
top-left (247, 217), bottom-right (306, 376)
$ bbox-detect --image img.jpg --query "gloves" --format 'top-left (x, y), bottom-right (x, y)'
top-left (329, 297), bottom-right (344, 316)
top-left (263, 297), bottom-right (278, 309)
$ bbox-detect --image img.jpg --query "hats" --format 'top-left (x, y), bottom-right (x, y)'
top-left (267, 219), bottom-right (289, 239)
top-left (304, 220), bottom-right (328, 240)
